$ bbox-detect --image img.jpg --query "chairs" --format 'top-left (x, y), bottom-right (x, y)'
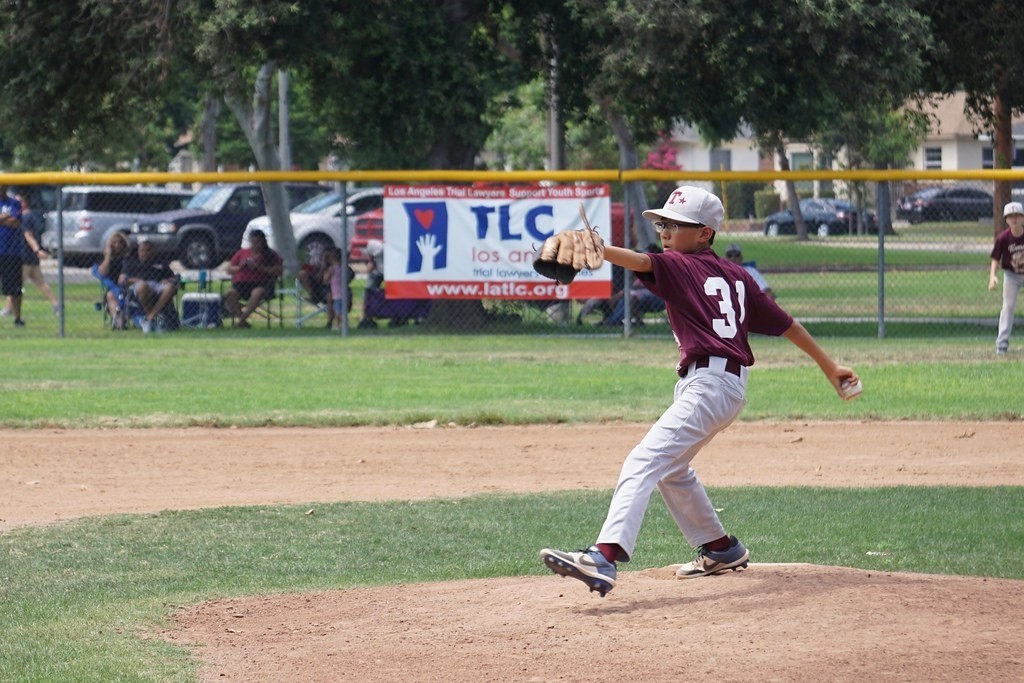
top-left (91, 263), bottom-right (328, 329)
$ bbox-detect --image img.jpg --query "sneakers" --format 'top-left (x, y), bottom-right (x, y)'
top-left (139, 316), bottom-right (156, 333)
top-left (540, 545), bottom-right (616, 598)
top-left (676, 536), bottom-right (749, 579)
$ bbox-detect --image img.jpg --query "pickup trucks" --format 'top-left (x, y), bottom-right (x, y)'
top-left (350, 179), bottom-right (638, 265)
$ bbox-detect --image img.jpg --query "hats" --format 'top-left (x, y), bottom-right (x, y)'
top-left (1003, 202), bottom-right (1024, 218)
top-left (726, 244), bottom-right (740, 253)
top-left (642, 186), bottom-right (724, 232)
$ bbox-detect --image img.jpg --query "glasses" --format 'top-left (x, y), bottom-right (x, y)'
top-left (654, 222), bottom-right (698, 233)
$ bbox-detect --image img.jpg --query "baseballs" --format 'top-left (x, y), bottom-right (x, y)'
top-left (841, 378), bottom-right (864, 401)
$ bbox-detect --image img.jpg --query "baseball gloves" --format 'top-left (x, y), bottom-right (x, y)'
top-left (532, 229), bottom-right (605, 285)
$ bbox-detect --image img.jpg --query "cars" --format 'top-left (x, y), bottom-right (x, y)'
top-left (241, 186), bottom-right (384, 267)
top-left (762, 198), bottom-right (877, 238)
top-left (129, 183), bottom-right (333, 269)
top-left (42, 186), bottom-right (197, 268)
top-left (896, 186), bottom-right (994, 224)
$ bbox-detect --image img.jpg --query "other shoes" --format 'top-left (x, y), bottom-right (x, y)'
top-left (997, 348), bottom-right (1007, 355)
top-left (0, 308), bottom-right (25, 326)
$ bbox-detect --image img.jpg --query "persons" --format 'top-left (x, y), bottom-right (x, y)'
top-left (988, 202), bottom-right (1024, 355)
top-left (92, 230), bottom-right (777, 336)
top-left (0, 183), bottom-right (63, 325)
top-left (532, 185), bottom-right (859, 597)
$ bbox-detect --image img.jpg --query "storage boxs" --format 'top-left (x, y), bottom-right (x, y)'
top-left (182, 292), bottom-right (223, 327)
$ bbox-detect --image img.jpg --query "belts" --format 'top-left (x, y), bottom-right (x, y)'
top-left (696, 356), bottom-right (740, 377)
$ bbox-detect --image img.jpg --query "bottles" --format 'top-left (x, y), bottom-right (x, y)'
top-left (199, 271), bottom-right (207, 288)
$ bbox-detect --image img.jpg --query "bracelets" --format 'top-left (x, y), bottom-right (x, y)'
top-left (34, 247), bottom-right (42, 255)
